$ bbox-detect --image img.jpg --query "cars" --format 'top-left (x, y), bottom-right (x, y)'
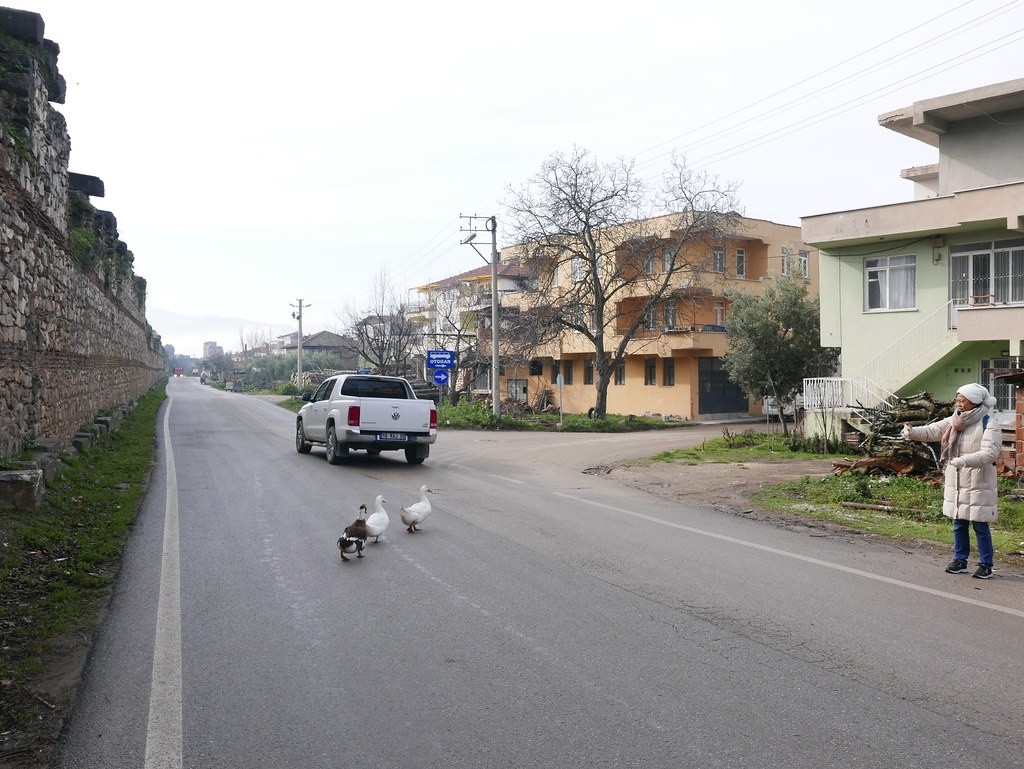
top-left (761, 384), bottom-right (805, 423)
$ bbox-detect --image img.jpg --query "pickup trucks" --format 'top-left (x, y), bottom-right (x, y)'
top-left (294, 370), bottom-right (437, 465)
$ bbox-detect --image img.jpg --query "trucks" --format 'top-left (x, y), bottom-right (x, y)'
top-left (172, 368), bottom-right (184, 378)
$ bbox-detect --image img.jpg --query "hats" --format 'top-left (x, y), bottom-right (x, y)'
top-left (957, 383), bottom-right (997, 407)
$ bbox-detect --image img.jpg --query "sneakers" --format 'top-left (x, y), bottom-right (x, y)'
top-left (971, 564), bottom-right (993, 579)
top-left (945, 557), bottom-right (968, 573)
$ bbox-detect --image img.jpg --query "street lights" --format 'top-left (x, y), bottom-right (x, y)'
top-left (460, 233), bottom-right (502, 420)
top-left (289, 299), bottom-right (312, 391)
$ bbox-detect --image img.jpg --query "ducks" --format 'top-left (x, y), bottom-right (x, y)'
top-left (400, 483), bottom-right (434, 535)
top-left (364, 495), bottom-right (390, 544)
top-left (336, 503), bottom-right (369, 560)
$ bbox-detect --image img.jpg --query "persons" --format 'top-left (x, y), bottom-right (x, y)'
top-left (900, 383), bottom-right (1002, 578)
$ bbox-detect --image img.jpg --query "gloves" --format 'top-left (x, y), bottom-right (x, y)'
top-left (903, 422), bottom-right (912, 436)
top-left (949, 457), bottom-right (965, 468)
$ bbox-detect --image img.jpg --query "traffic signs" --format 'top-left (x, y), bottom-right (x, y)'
top-left (426, 349), bottom-right (455, 371)
